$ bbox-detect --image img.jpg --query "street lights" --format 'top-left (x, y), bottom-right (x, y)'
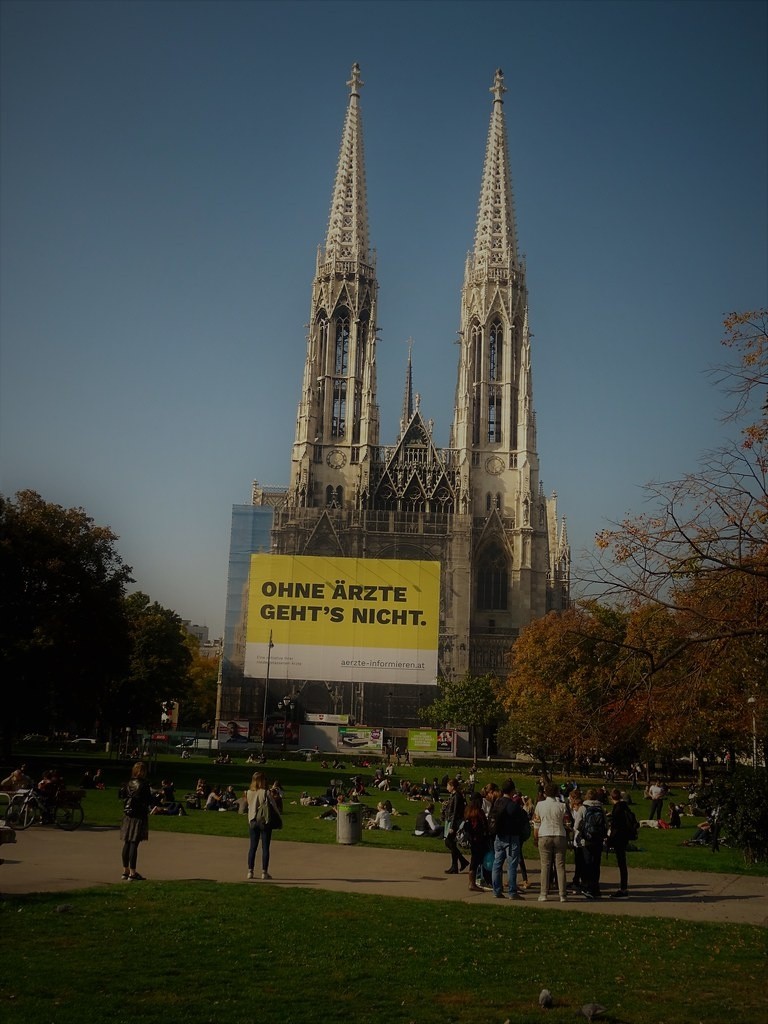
top-left (748, 696), bottom-right (757, 768)
top-left (260, 628), bottom-right (275, 755)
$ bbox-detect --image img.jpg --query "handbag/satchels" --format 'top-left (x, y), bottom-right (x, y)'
top-left (257, 790), bottom-right (282, 829)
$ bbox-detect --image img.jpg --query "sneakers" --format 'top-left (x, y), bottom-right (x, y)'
top-left (127, 872), bottom-right (146, 881)
top-left (122, 872), bottom-right (129, 879)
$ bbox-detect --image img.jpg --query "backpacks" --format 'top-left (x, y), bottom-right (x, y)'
top-left (488, 799), bottom-right (512, 834)
top-left (622, 806), bottom-right (640, 841)
top-left (581, 805), bottom-right (606, 844)
top-left (457, 819), bottom-right (474, 848)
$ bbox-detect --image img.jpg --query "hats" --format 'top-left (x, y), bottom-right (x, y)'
top-left (469, 792), bottom-right (486, 800)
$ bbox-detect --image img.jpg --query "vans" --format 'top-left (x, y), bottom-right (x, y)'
top-left (71, 738), bottom-right (98, 743)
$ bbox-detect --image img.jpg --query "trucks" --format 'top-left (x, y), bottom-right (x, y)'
top-left (175, 739), bottom-right (221, 750)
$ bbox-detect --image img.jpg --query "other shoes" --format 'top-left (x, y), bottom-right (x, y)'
top-left (219, 807), bottom-right (226, 812)
top-left (469, 887), bottom-right (483, 891)
top-left (445, 866), bottom-right (458, 874)
top-left (565, 883), bottom-right (579, 893)
top-left (560, 895), bottom-right (569, 901)
top-left (609, 890), bottom-right (629, 899)
top-left (509, 894), bottom-right (525, 900)
top-left (523, 880), bottom-right (530, 888)
top-left (504, 880), bottom-right (510, 885)
top-left (494, 895), bottom-right (505, 898)
top-left (460, 862), bottom-right (469, 871)
top-left (262, 873), bottom-right (272, 879)
top-left (581, 890), bottom-right (594, 899)
top-left (538, 896), bottom-right (546, 901)
top-left (247, 872), bottom-right (254, 879)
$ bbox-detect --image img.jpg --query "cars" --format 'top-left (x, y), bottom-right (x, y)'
top-left (241, 747), bottom-right (260, 753)
top-left (288, 748), bottom-right (321, 755)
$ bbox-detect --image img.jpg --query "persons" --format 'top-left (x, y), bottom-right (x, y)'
top-left (0, 697), bottom-right (768, 901)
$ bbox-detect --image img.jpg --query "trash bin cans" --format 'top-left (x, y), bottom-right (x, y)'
top-left (336, 802), bottom-right (362, 845)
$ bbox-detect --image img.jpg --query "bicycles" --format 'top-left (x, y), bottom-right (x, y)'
top-left (5, 782), bottom-right (85, 831)
